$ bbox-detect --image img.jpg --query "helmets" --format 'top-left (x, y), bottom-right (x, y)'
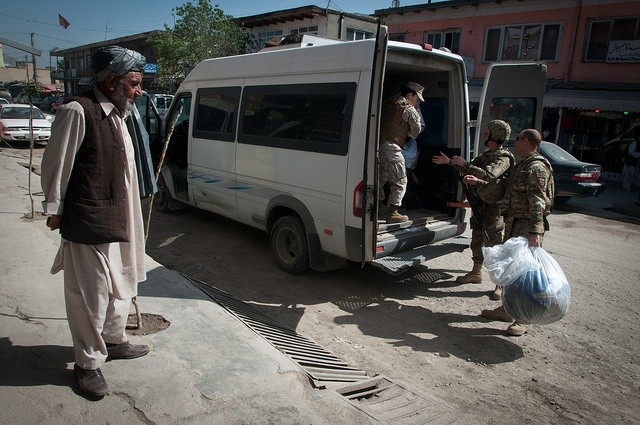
top-left (488, 119), bottom-right (512, 142)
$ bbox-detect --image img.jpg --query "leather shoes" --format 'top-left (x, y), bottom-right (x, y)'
top-left (106, 340), bottom-right (150, 361)
top-left (74, 362), bottom-right (109, 397)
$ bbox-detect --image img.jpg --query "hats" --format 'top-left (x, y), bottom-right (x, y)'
top-left (405, 81), bottom-right (425, 102)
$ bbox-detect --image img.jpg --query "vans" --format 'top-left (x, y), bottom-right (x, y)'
top-left (134, 23), bottom-right (547, 278)
top-left (139, 93), bottom-right (174, 118)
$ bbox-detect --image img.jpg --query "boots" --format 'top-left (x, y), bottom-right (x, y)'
top-left (456, 261), bottom-right (484, 284)
top-left (482, 305), bottom-right (514, 322)
top-left (386, 204), bottom-right (409, 223)
top-left (507, 321), bottom-right (533, 336)
top-left (491, 285), bottom-right (503, 301)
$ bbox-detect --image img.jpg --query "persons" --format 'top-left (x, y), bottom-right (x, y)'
top-left (378, 81), bottom-right (425, 223)
top-left (463, 128), bottom-right (555, 335)
top-left (40, 45), bottom-right (159, 398)
top-left (430, 119), bottom-right (516, 301)
top-left (621, 135), bottom-right (640, 191)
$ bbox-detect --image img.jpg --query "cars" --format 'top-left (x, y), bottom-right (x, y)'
top-left (504, 137), bottom-right (606, 201)
top-left (1, 104), bottom-right (51, 144)
